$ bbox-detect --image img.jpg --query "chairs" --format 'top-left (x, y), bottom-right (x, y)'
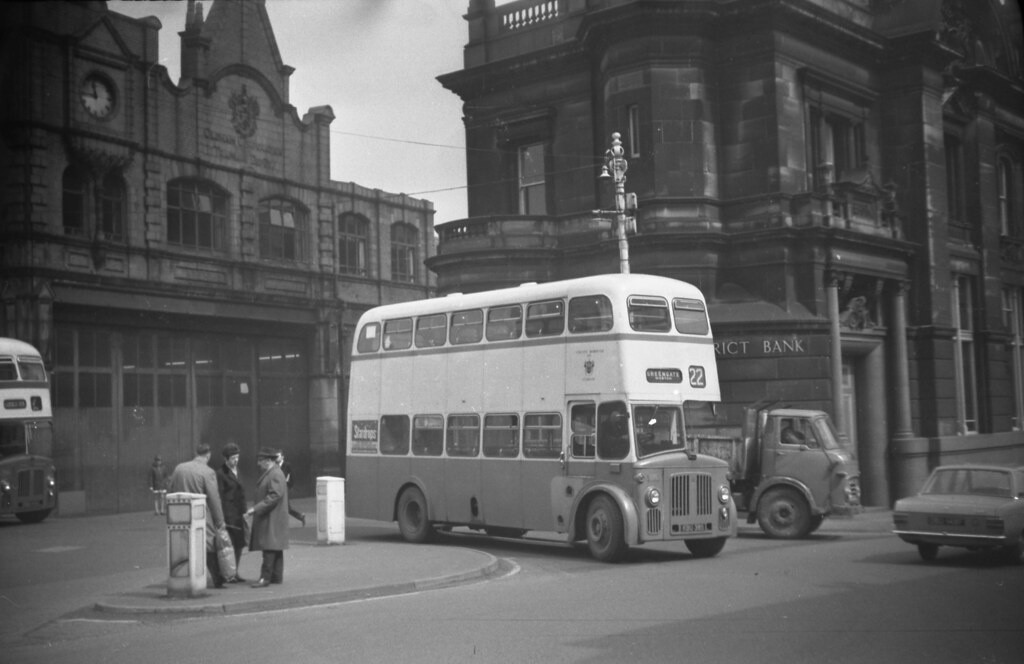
top-left (380, 443), bottom-right (563, 458)
top-left (385, 323), bottom-right (579, 351)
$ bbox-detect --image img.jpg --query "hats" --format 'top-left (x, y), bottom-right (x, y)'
top-left (256, 447), bottom-right (279, 457)
top-left (222, 443), bottom-right (240, 456)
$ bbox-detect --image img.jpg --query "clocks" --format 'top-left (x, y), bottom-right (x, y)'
top-left (80, 69), bottom-right (122, 121)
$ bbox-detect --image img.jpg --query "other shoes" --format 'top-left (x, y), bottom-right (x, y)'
top-left (251, 577), bottom-right (268, 588)
top-left (235, 575), bottom-right (245, 581)
top-left (301, 514), bottom-right (305, 527)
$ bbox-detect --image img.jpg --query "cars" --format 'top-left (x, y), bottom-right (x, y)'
top-left (894, 463), bottom-right (1024, 564)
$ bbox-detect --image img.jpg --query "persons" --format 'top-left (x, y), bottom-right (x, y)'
top-left (246, 448), bottom-right (288, 586)
top-left (149, 456), bottom-right (168, 515)
top-left (276, 448), bottom-right (305, 526)
top-left (601, 410), bottom-right (624, 456)
top-left (217, 443), bottom-right (250, 582)
top-left (783, 420), bottom-right (804, 444)
top-left (168, 443), bottom-right (225, 589)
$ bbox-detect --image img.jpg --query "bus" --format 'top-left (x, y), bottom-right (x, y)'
top-left (344, 274), bottom-right (738, 563)
top-left (0, 337), bottom-right (57, 523)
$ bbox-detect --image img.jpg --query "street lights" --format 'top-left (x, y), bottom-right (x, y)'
top-left (592, 132), bottom-right (637, 274)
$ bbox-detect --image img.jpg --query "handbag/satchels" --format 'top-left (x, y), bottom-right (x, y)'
top-left (216, 523), bottom-right (236, 582)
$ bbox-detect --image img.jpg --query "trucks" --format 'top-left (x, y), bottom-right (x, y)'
top-left (676, 408), bottom-right (864, 539)
